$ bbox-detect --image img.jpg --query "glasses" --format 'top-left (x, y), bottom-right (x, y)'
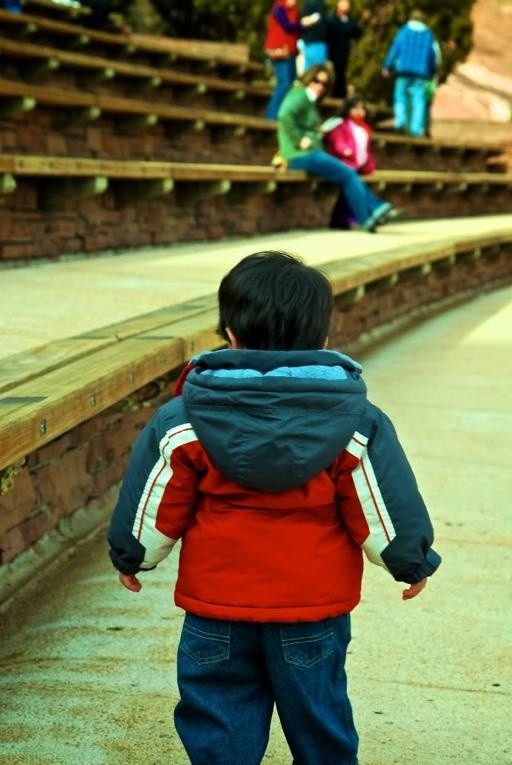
top-left (315, 79), bottom-right (328, 87)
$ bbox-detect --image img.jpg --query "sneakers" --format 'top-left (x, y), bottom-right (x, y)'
top-left (365, 203), bottom-right (392, 231)
top-left (377, 207), bottom-right (401, 224)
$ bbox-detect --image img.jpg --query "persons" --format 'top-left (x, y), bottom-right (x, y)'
top-left (380, 8), bottom-right (441, 138)
top-left (107, 249), bottom-right (443, 765)
top-left (264, 0), bottom-right (392, 232)
